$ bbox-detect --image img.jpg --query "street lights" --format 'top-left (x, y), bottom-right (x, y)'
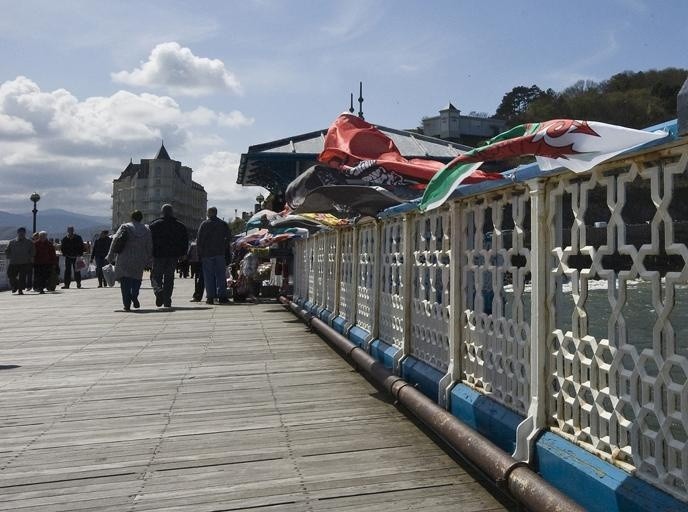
top-left (30, 192), bottom-right (40, 233)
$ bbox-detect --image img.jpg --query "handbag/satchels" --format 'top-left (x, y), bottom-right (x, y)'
top-left (102, 263), bottom-right (116, 287)
top-left (112, 229), bottom-right (128, 254)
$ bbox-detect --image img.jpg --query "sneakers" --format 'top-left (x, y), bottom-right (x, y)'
top-left (122, 289), bottom-right (172, 311)
top-left (189, 296), bottom-right (234, 305)
top-left (10, 282), bottom-right (82, 294)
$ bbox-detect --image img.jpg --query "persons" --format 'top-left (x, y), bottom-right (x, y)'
top-left (150, 203), bottom-right (188, 307)
top-left (61, 226), bottom-right (84, 289)
top-left (177, 207), bottom-right (231, 304)
top-left (5, 227), bottom-right (58, 295)
top-left (105, 210), bottom-right (153, 311)
top-left (90, 230), bottom-right (113, 287)
